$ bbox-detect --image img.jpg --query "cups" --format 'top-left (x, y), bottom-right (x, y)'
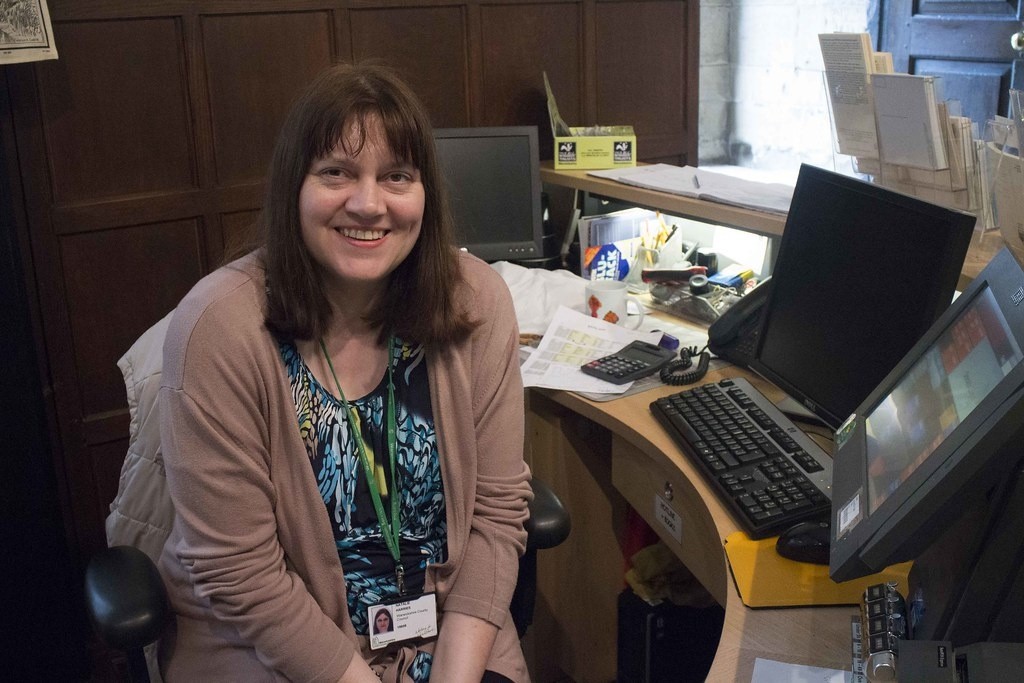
top-left (585, 280), bottom-right (645, 330)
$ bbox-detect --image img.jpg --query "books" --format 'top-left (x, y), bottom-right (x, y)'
top-left (617, 163), bottom-right (795, 217)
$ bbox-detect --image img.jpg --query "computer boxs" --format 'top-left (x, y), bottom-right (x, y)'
top-left (617, 587), bottom-right (725, 683)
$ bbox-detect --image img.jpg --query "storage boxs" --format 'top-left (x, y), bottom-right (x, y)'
top-left (618, 591), bottom-right (727, 683)
top-left (553, 127), bottom-right (638, 171)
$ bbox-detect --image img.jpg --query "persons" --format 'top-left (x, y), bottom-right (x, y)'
top-left (373, 608), bottom-right (394, 634)
top-left (163, 60), bottom-right (534, 683)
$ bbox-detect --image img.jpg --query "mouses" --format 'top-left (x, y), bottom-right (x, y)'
top-left (775, 519), bottom-right (830, 565)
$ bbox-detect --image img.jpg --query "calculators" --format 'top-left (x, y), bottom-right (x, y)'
top-left (582, 339), bottom-right (678, 385)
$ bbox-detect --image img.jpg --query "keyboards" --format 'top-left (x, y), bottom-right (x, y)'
top-left (649, 376), bottom-right (833, 541)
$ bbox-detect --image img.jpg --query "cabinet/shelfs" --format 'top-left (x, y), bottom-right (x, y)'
top-left (512, 163), bottom-right (1024, 683)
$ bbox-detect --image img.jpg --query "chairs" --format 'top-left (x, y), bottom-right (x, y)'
top-left (84, 473), bottom-right (573, 683)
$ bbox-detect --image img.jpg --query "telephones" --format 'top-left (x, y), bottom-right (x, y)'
top-left (707, 275), bottom-right (777, 367)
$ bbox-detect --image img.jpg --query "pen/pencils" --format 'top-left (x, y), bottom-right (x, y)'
top-left (694, 173), bottom-right (702, 189)
top-left (640, 210), bottom-right (678, 266)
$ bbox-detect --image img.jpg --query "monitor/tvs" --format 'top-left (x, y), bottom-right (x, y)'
top-left (829, 243), bottom-right (1024, 583)
top-left (431, 125), bottom-right (544, 261)
top-left (747, 163), bottom-right (977, 432)
